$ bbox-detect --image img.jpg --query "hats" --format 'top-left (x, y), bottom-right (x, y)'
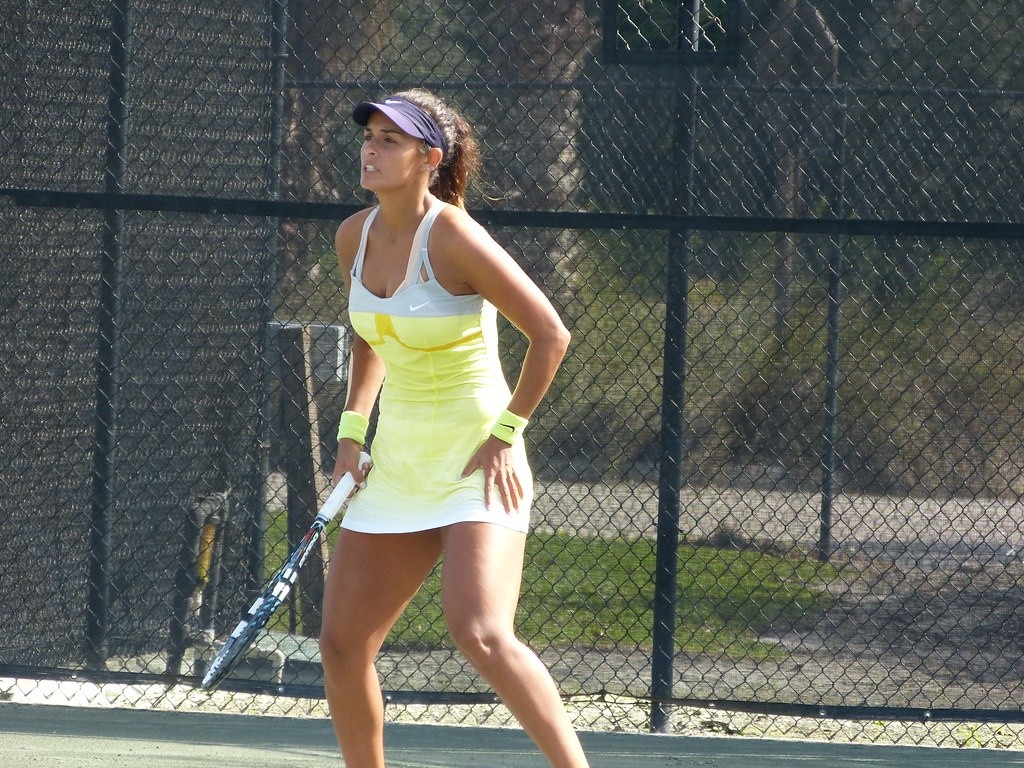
top-left (353, 88), bottom-right (448, 165)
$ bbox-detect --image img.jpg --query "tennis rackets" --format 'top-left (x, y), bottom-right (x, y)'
top-left (200, 450), bottom-right (373, 694)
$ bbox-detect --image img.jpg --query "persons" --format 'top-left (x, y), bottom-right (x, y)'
top-left (317, 87), bottom-right (591, 768)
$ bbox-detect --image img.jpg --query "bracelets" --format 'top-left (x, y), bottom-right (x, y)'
top-left (492, 409), bottom-right (530, 445)
top-left (337, 411), bottom-right (369, 445)
